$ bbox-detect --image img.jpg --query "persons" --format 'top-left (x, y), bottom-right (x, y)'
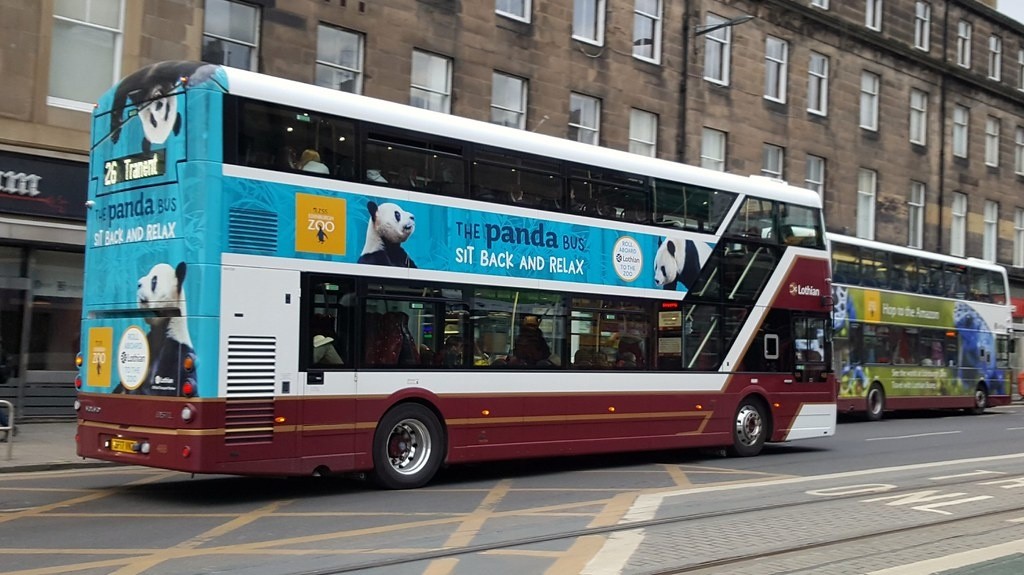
top-left (277, 144), bottom-right (302, 172)
top-left (780, 211), bottom-right (795, 241)
top-left (568, 186), bottom-right (590, 217)
top-left (297, 148), bottom-right (332, 174)
top-left (507, 183), bottom-right (526, 207)
top-left (312, 333), bottom-right (344, 366)
top-left (920, 352), bottom-right (935, 366)
top-left (421, 320), bottom-right (649, 372)
top-left (366, 159), bottom-right (390, 183)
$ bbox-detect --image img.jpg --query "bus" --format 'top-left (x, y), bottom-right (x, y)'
top-left (761, 223), bottom-right (1016, 421)
top-left (72, 57), bottom-right (836, 490)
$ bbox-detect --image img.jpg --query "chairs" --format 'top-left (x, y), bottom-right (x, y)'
top-left (833, 257), bottom-right (1000, 304)
top-left (309, 292), bottom-right (658, 372)
top-left (226, 130), bottom-right (759, 238)
